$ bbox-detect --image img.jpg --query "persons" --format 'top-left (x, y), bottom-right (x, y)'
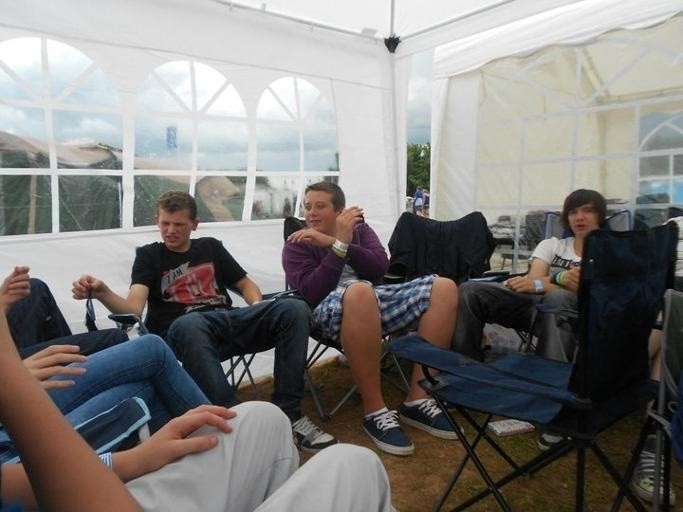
top-left (0, 266), bottom-right (217, 451)
top-left (421, 189), bottom-right (429, 219)
top-left (629, 217), bottom-right (682, 507)
top-left (70, 189), bottom-right (341, 454)
top-left (437, 188), bottom-right (606, 454)
top-left (412, 185), bottom-right (423, 218)
top-left (0, 301), bottom-right (400, 512)
top-left (280, 182), bottom-right (466, 457)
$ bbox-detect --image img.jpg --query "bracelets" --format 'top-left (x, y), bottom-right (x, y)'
top-left (331, 241), bottom-right (349, 257)
top-left (532, 279), bottom-right (545, 295)
top-left (96, 453), bottom-right (112, 470)
top-left (550, 270), bottom-right (567, 286)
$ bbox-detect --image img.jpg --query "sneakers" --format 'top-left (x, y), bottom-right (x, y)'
top-left (291, 415), bottom-right (340, 455)
top-left (538, 432), bottom-right (564, 451)
top-left (361, 410), bottom-right (415, 456)
top-left (631, 435), bottom-right (676, 505)
top-left (398, 398), bottom-right (465, 440)
